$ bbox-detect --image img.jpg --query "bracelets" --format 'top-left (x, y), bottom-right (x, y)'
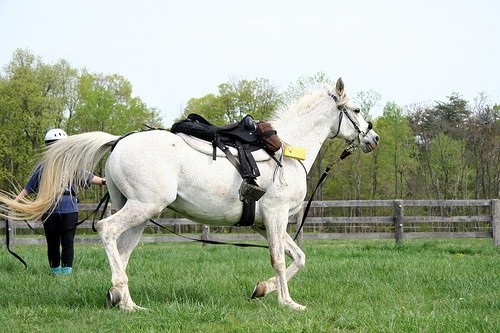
top-left (102, 178), bottom-right (106, 186)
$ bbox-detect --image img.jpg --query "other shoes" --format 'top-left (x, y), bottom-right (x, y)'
top-left (50, 267), bottom-right (61, 276)
top-left (62, 268), bottom-right (73, 276)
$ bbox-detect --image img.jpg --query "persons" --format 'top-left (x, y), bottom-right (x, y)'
top-left (14, 128), bottom-right (106, 275)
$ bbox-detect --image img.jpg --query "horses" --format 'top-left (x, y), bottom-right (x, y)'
top-left (0, 77), bottom-right (380, 312)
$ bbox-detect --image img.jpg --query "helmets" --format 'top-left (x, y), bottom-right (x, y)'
top-left (44, 128), bottom-right (68, 143)
